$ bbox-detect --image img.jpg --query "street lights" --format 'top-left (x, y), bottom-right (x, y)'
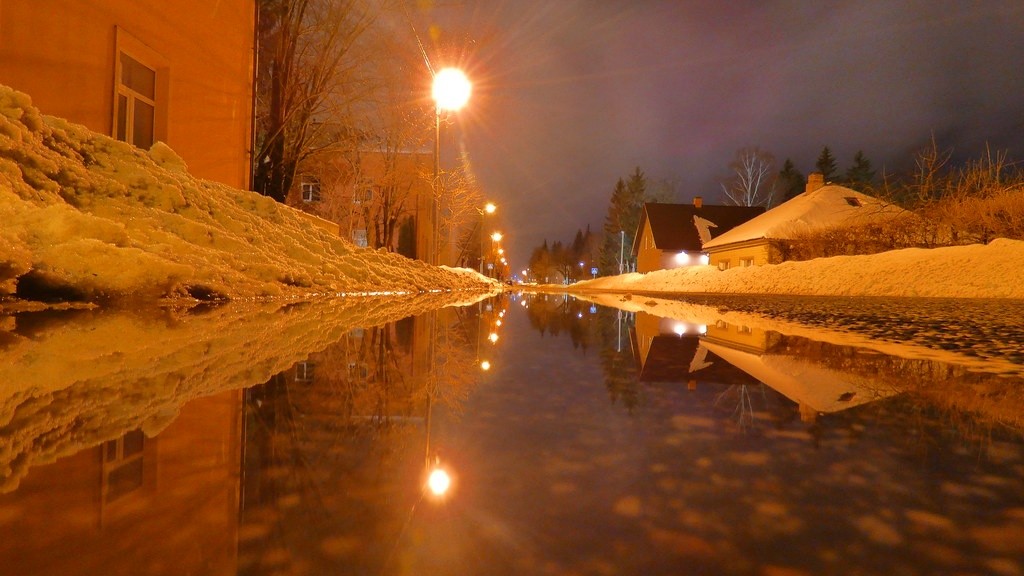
top-left (479, 201), bottom-right (497, 275)
top-left (476, 289), bottom-right (491, 371)
top-left (424, 306), bottom-right (453, 495)
top-left (429, 64), bottom-right (473, 265)
top-left (489, 232), bottom-right (530, 288)
